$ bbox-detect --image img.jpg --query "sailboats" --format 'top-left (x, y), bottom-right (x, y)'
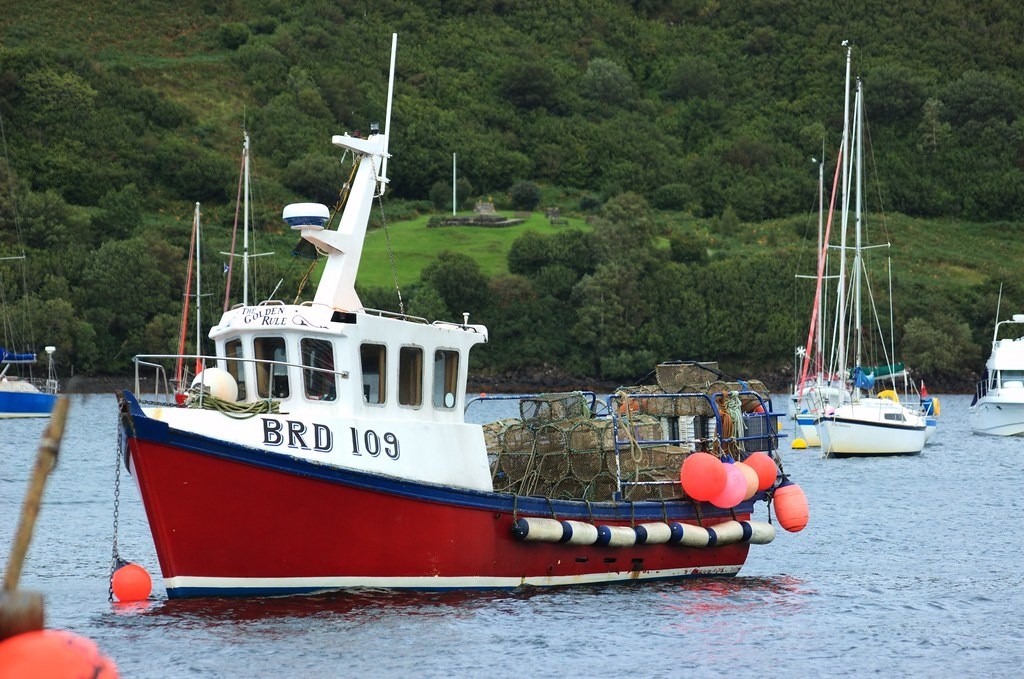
top-left (788, 41), bottom-right (945, 459)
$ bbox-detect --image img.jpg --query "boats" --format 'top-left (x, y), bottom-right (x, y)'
top-left (0, 117), bottom-right (64, 421)
top-left (108, 31), bottom-right (811, 612)
top-left (966, 279), bottom-right (1024, 440)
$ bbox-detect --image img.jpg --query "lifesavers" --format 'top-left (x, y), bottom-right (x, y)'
top-left (878, 389), bottom-right (899, 403)
